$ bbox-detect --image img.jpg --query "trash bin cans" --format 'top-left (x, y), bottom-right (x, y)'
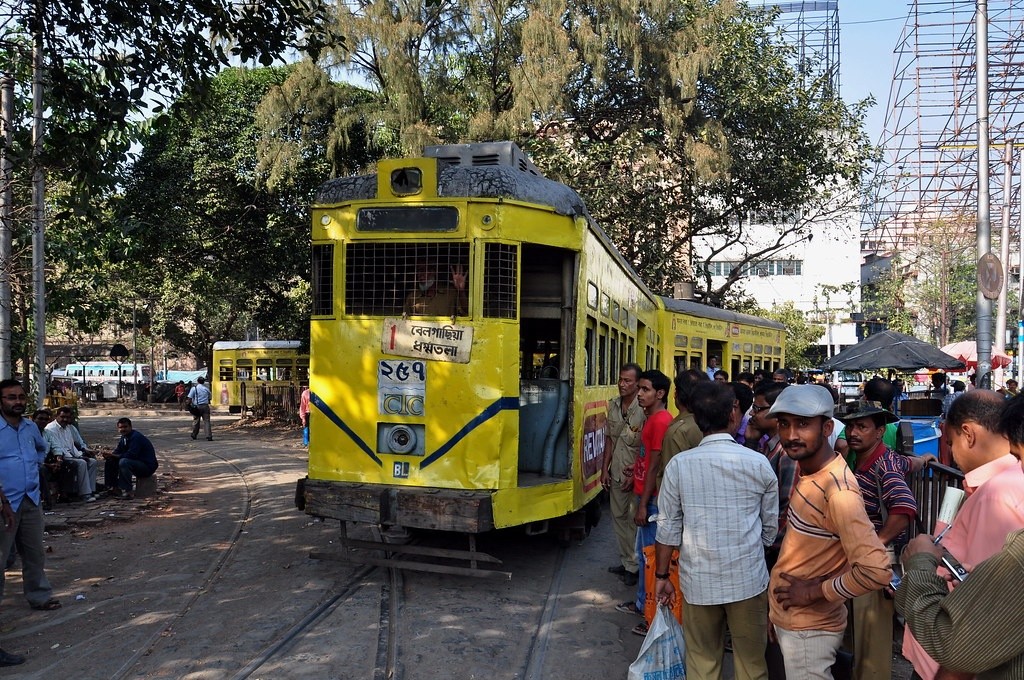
top-left (900, 417), bottom-right (940, 479)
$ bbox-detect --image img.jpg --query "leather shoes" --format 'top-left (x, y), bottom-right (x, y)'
top-left (0, 649), bottom-right (26, 667)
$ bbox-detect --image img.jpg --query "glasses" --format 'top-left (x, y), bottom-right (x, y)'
top-left (753, 404), bottom-right (771, 413)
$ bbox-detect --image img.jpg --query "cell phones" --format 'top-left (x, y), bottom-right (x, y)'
top-left (941, 547), bottom-right (969, 582)
top-left (890, 572), bottom-right (901, 591)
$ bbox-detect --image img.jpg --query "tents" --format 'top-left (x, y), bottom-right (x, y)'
top-left (817, 329), bottom-right (966, 381)
top-left (940, 341), bottom-right (1012, 372)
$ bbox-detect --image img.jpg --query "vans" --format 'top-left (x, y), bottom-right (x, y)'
top-left (829, 370), bottom-right (865, 399)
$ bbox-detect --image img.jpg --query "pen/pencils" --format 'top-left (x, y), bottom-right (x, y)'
top-left (934, 525), bottom-right (953, 544)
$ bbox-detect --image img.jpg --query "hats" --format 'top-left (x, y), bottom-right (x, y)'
top-left (764, 384), bottom-right (835, 419)
top-left (950, 381), bottom-right (966, 388)
top-left (834, 399), bottom-right (900, 425)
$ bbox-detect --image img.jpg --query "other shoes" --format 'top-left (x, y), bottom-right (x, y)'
top-left (206, 436), bottom-right (213, 441)
top-left (190, 434), bottom-right (197, 440)
top-left (114, 490), bottom-right (134, 499)
top-left (624, 572), bottom-right (637, 586)
top-left (92, 491), bottom-right (100, 498)
top-left (607, 564), bottom-right (624, 575)
top-left (79, 494), bottom-right (96, 502)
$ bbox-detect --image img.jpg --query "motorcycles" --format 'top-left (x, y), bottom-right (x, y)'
top-left (891, 373), bottom-right (951, 478)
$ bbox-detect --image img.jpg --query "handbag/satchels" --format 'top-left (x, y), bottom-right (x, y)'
top-left (188, 404), bottom-right (199, 416)
top-left (643, 544), bottom-right (682, 629)
top-left (627, 601), bottom-right (687, 680)
top-left (303, 427), bottom-right (308, 445)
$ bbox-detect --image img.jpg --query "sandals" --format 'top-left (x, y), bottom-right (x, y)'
top-left (31, 598), bottom-right (60, 610)
top-left (615, 600), bottom-right (645, 617)
top-left (632, 620), bottom-right (649, 636)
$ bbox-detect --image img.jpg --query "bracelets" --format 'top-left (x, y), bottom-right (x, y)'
top-left (654, 571), bottom-right (669, 578)
top-left (2, 500), bottom-right (9, 504)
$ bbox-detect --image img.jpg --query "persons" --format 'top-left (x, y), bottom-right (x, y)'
top-left (0, 379), bottom-right (62, 610)
top-left (403, 257), bottom-right (469, 316)
top-left (601, 355), bottom-right (1024, 680)
top-left (99, 417), bottom-right (158, 500)
top-left (299, 389), bottom-right (310, 443)
top-left (175, 380), bottom-right (194, 411)
top-left (46, 380), bottom-right (76, 406)
top-left (187, 377), bottom-right (212, 441)
top-left (32, 406), bottom-right (100, 503)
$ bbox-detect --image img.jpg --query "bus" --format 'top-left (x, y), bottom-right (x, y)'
top-left (210, 340), bottom-right (311, 412)
top-left (63, 361), bottom-right (157, 399)
top-left (294, 141), bottom-right (661, 581)
top-left (653, 295), bottom-right (786, 420)
